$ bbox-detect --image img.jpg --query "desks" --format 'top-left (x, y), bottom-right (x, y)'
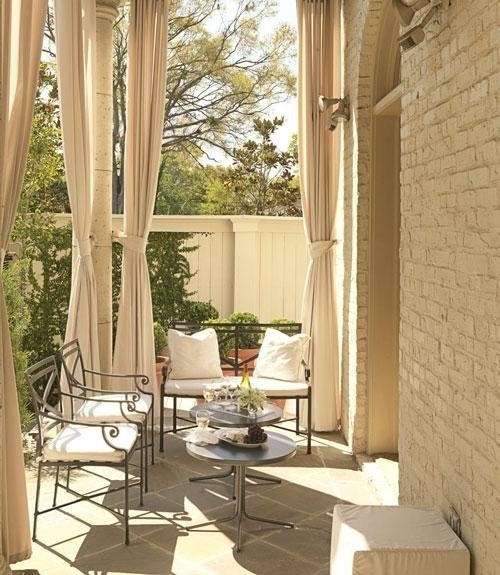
top-left (186, 430), bottom-right (296, 553)
top-left (189, 402), bottom-right (283, 500)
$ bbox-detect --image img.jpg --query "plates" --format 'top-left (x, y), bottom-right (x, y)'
top-left (213, 427), bottom-right (272, 449)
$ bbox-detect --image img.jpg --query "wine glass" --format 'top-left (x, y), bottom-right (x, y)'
top-left (201, 376), bottom-right (239, 410)
top-left (194, 409), bottom-right (211, 447)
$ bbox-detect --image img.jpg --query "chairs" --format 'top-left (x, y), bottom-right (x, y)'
top-left (24, 355), bottom-right (144, 545)
top-left (57, 338), bottom-right (154, 493)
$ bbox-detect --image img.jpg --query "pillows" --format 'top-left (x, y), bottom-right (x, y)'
top-left (253, 329), bottom-right (311, 382)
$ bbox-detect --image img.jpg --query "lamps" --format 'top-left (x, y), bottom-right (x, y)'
top-left (319, 95), bottom-right (350, 132)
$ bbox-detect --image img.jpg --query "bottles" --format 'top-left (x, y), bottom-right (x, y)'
top-left (239, 362), bottom-right (252, 390)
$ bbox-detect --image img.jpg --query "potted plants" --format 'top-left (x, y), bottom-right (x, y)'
top-left (154, 312), bottom-right (260, 391)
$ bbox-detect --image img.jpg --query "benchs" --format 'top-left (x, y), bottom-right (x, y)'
top-left (160, 322), bottom-right (311, 455)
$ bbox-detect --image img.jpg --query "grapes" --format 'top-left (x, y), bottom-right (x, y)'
top-left (244, 422), bottom-right (267, 443)
